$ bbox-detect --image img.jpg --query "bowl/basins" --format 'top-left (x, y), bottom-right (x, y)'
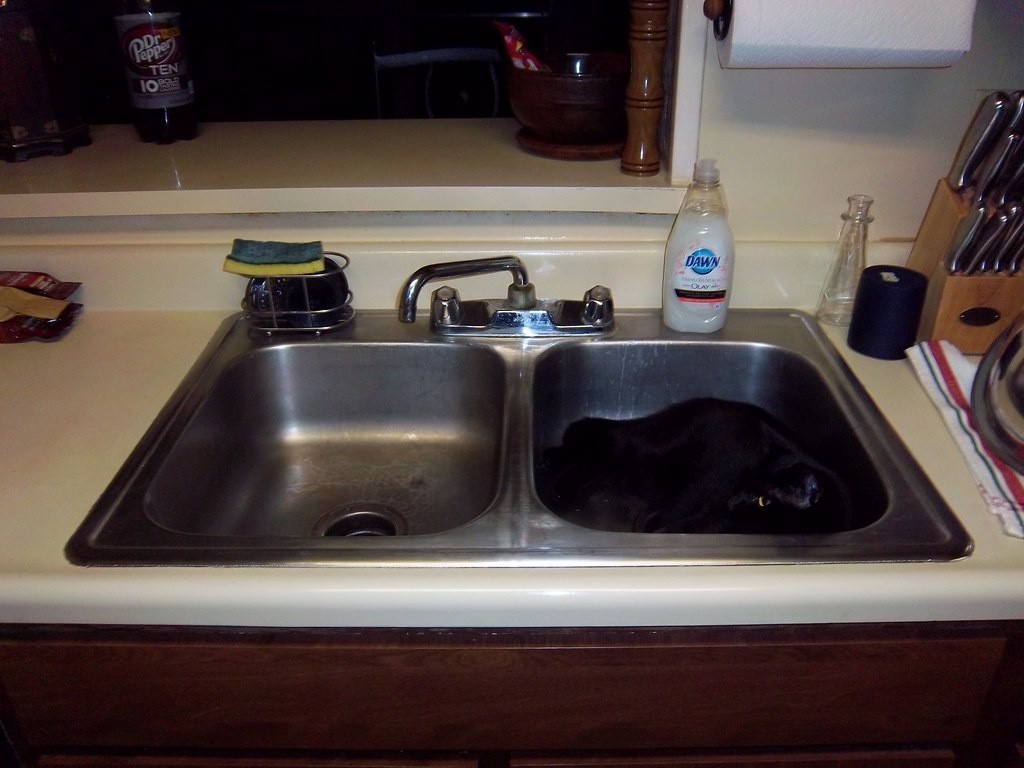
top-left (507, 52), bottom-right (631, 143)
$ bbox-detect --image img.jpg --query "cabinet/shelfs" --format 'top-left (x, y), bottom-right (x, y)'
top-left (0, 619), bottom-right (1024, 767)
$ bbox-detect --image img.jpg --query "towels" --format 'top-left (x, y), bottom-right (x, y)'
top-left (904, 338), bottom-right (1024, 540)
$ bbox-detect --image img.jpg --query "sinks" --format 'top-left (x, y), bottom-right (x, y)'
top-left (63, 308), bottom-right (527, 566)
top-left (517, 307), bottom-right (973, 565)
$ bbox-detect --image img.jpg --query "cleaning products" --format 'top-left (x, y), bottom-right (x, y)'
top-left (661, 156), bottom-right (736, 335)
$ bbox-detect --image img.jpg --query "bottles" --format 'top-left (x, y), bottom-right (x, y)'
top-left (816, 195), bottom-right (873, 327)
top-left (113, 0), bottom-right (198, 144)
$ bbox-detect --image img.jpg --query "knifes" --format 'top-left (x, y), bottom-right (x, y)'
top-left (947, 89), bottom-right (1024, 276)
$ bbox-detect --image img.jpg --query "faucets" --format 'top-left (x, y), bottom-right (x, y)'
top-left (398, 256), bottom-right (616, 339)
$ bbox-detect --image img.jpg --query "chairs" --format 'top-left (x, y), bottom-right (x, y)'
top-left (371, 48), bottom-right (505, 116)
